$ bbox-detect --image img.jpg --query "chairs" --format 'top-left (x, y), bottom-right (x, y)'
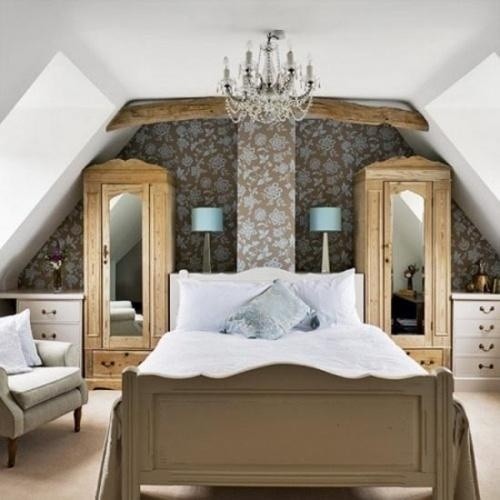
top-left (0, 340), bottom-right (88, 467)
top-left (109, 301), bottom-right (142, 336)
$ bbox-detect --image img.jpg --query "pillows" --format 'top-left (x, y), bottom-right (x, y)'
top-left (174, 277), bottom-right (274, 331)
top-left (220, 279), bottom-right (316, 340)
top-left (0, 308), bottom-right (42, 367)
top-left (0, 313), bottom-right (33, 375)
top-left (255, 268), bottom-right (366, 330)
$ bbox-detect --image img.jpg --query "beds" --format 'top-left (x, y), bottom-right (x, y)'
top-left (95, 269), bottom-right (480, 500)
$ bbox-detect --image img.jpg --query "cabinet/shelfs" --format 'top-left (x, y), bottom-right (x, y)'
top-left (353, 156), bottom-right (451, 371)
top-left (451, 293), bottom-right (500, 392)
top-left (84, 159), bottom-right (174, 391)
top-left (0, 290), bottom-right (85, 367)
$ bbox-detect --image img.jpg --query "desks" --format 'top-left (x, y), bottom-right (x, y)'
top-left (392, 291), bottom-right (424, 333)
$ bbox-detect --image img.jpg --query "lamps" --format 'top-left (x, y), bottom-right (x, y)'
top-left (218, 29), bottom-right (319, 125)
top-left (309, 207), bottom-right (342, 273)
top-left (191, 207), bottom-right (223, 272)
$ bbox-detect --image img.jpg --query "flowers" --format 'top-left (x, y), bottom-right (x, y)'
top-left (45, 245), bottom-right (72, 287)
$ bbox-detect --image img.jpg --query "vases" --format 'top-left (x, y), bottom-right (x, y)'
top-left (53, 270), bottom-right (62, 292)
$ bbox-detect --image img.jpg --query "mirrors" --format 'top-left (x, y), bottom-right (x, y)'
top-left (109, 193), bottom-right (142, 336)
top-left (391, 189), bottom-right (427, 335)
